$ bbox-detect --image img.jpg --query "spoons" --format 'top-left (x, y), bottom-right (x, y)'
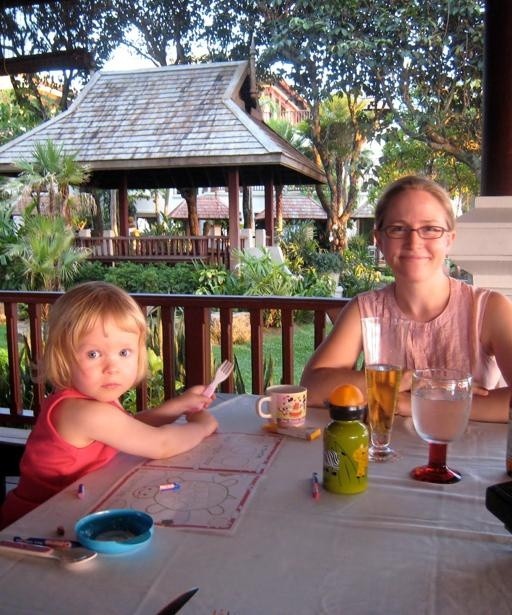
top-left (0, 540), bottom-right (97, 567)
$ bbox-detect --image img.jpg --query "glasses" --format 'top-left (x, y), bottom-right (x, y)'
top-left (377, 223), bottom-right (451, 240)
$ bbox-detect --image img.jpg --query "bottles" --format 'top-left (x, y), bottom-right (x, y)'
top-left (321, 385), bottom-right (370, 494)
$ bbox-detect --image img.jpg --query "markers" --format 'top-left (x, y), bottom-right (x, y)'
top-left (159, 484), bottom-right (180, 490)
top-left (312, 472), bottom-right (320, 500)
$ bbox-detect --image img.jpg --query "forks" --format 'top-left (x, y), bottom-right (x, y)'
top-left (193, 360), bottom-right (235, 410)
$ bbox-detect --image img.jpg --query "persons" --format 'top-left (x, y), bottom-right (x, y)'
top-left (301, 174), bottom-right (511, 428)
top-left (1, 281), bottom-right (218, 534)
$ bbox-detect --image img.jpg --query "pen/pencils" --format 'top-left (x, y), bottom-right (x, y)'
top-left (14, 537), bottom-right (81, 547)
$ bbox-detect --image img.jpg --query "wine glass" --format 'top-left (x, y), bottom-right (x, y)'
top-left (409, 368), bottom-right (475, 485)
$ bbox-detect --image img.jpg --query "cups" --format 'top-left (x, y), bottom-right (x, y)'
top-left (360, 317), bottom-right (411, 465)
top-left (256, 385), bottom-right (307, 430)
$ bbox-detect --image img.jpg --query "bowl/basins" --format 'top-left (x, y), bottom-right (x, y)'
top-left (103, 230), bottom-right (116, 239)
top-left (76, 508), bottom-right (154, 554)
top-left (78, 228), bottom-right (91, 237)
